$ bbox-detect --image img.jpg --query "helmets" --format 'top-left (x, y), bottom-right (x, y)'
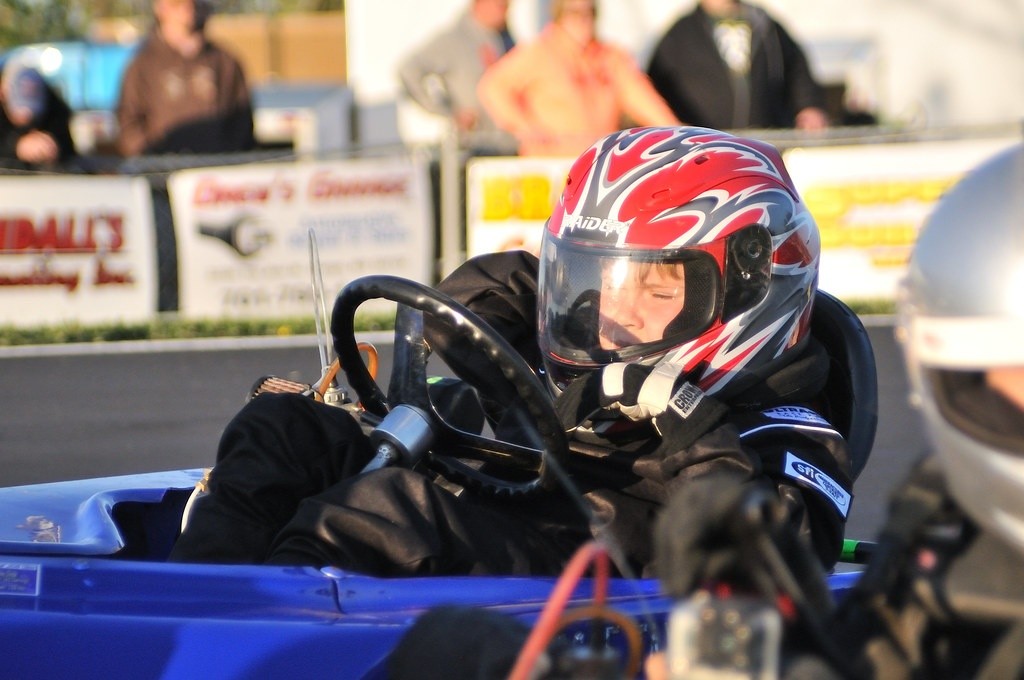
top-left (901, 144), bottom-right (1024, 558)
top-left (534, 127), bottom-right (824, 444)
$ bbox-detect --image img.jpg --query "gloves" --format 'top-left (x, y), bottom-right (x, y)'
top-left (652, 476), bottom-right (787, 596)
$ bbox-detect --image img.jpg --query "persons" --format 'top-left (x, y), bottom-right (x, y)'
top-left (0, 63), bottom-right (77, 163)
top-left (396, 0), bottom-right (522, 157)
top-left (777, 138), bottom-right (1023, 680)
top-left (647, 0), bottom-right (830, 136)
top-left (115, 0), bottom-right (257, 155)
top-left (475, 0), bottom-right (683, 158)
top-left (168, 128), bottom-right (854, 579)
